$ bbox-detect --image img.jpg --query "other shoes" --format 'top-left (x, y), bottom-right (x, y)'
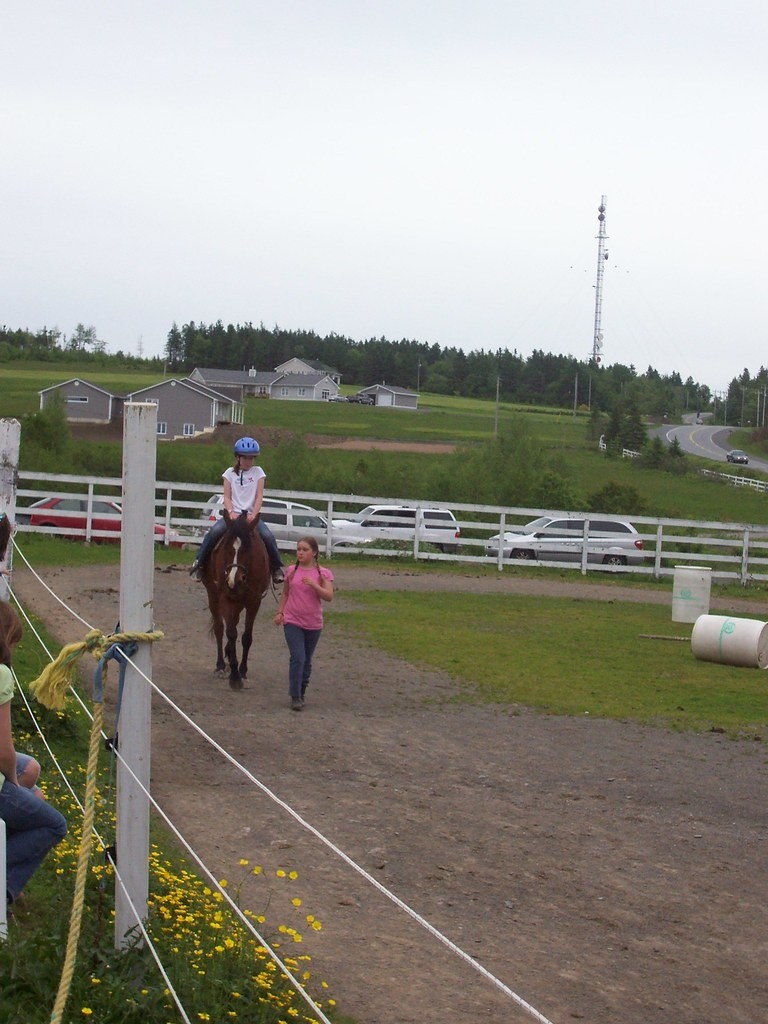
top-left (273, 569), bottom-right (284, 582)
top-left (190, 562), bottom-right (202, 581)
top-left (292, 697), bottom-right (305, 708)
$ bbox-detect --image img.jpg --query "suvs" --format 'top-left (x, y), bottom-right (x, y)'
top-left (328, 504), bottom-right (464, 556)
top-left (194, 493), bottom-right (359, 552)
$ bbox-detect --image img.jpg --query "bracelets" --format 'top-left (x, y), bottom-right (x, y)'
top-left (228, 510), bottom-right (233, 514)
top-left (277, 612), bottom-right (284, 616)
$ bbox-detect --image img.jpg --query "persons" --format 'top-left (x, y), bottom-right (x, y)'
top-left (190, 437), bottom-right (284, 583)
top-left (15, 751), bottom-right (45, 801)
top-left (0, 512), bottom-right (14, 577)
top-left (0, 598), bottom-right (66, 914)
top-left (273, 536), bottom-right (334, 710)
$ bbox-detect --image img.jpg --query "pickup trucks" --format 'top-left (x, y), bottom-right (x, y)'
top-left (347, 393), bottom-right (375, 405)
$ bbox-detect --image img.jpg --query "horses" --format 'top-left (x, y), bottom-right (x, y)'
top-left (200, 507), bottom-right (271, 690)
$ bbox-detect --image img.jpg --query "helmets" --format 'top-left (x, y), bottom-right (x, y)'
top-left (235, 437), bottom-right (260, 456)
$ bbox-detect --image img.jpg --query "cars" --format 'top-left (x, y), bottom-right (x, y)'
top-left (726, 449), bottom-right (749, 464)
top-left (328, 394), bottom-right (350, 403)
top-left (484, 516), bottom-right (647, 573)
top-left (18, 495), bottom-right (186, 552)
